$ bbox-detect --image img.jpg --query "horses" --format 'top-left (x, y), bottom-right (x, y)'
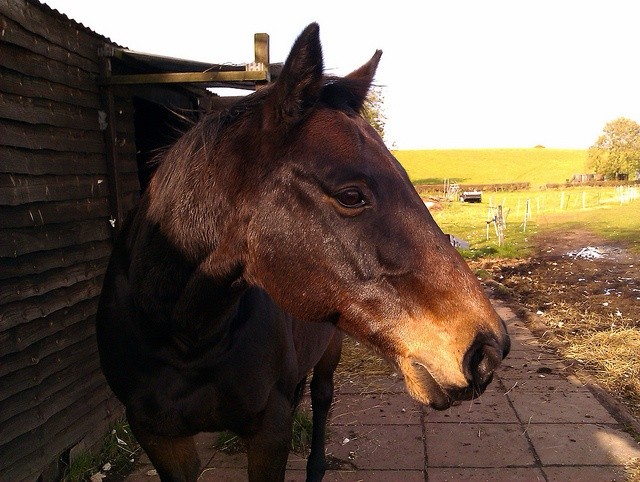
top-left (97, 21), bottom-right (511, 482)
